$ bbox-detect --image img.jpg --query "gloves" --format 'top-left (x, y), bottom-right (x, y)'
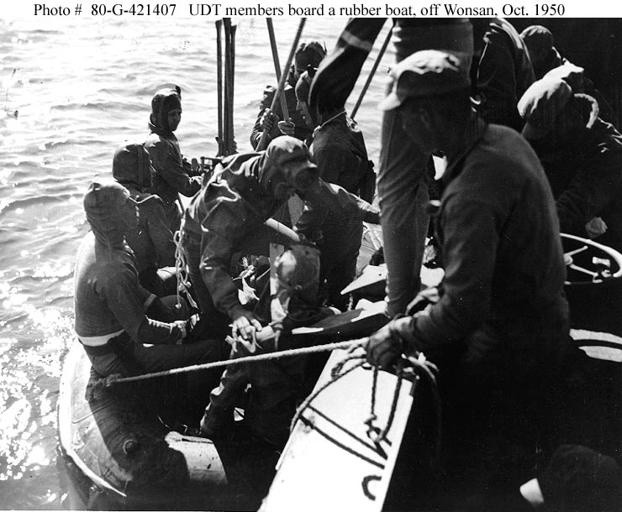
top-left (365, 316), bottom-right (428, 371)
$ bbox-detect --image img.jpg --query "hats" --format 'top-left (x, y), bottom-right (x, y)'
top-left (516, 77), bottom-right (573, 142)
top-left (378, 48), bottom-right (469, 111)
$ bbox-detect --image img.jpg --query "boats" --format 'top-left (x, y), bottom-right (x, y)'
top-left (57, 146), bottom-right (622, 512)
